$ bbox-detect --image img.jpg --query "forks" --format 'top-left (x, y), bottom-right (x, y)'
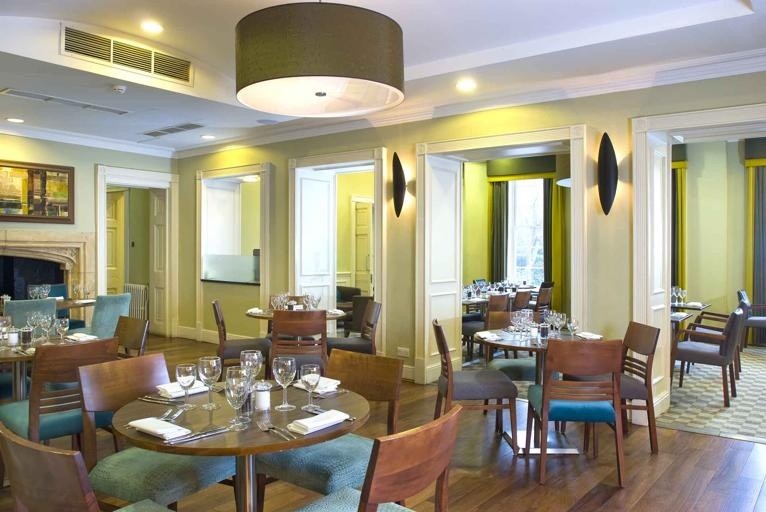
top-left (122, 408), bottom-right (183, 430)
top-left (254, 421), bottom-right (290, 442)
top-left (261, 418), bottom-right (297, 441)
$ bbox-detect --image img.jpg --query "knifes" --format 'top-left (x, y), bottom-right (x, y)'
top-left (10, 348), bottom-right (29, 356)
top-left (301, 407), bottom-right (356, 421)
top-left (311, 390), bottom-right (347, 399)
top-left (136, 395), bottom-right (179, 406)
top-left (163, 427), bottom-right (232, 446)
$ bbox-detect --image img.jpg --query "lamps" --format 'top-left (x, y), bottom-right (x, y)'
top-left (392, 151), bottom-right (408, 217)
top-left (232, 1), bottom-right (407, 120)
top-left (597, 131), bottom-right (620, 216)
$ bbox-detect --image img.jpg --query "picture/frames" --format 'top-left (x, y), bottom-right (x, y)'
top-left (0, 158), bottom-right (75, 224)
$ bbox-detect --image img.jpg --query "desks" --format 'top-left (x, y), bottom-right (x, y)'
top-left (111, 380), bottom-right (369, 511)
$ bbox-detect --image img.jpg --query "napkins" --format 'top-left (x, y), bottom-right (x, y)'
top-left (128, 417), bottom-right (191, 441)
top-left (155, 379), bottom-right (208, 399)
top-left (286, 408), bottom-right (350, 436)
top-left (294, 374), bottom-right (341, 394)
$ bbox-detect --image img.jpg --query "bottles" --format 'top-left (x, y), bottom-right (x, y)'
top-left (252, 381), bottom-right (271, 412)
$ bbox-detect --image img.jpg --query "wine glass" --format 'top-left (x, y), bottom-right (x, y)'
top-left (462, 279), bottom-right (522, 301)
top-left (26, 310), bottom-right (70, 346)
top-left (28, 282), bottom-right (52, 301)
top-left (175, 362), bottom-right (195, 412)
top-left (270, 290), bottom-right (323, 312)
top-left (223, 350), bottom-right (263, 432)
top-left (197, 356), bottom-right (223, 412)
top-left (198, 410), bottom-right (225, 444)
top-left (272, 356), bottom-right (297, 413)
top-left (509, 307), bottom-right (579, 343)
top-left (299, 364), bottom-right (320, 411)
top-left (82, 287), bottom-right (89, 299)
top-left (670, 286), bottom-right (687, 306)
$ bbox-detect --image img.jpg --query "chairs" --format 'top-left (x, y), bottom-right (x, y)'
top-left (432, 319), bottom-right (519, 453)
top-left (523, 338), bottom-right (626, 485)
top-left (296, 405), bottom-right (463, 510)
top-left (2, 279), bottom-right (149, 404)
top-left (2, 337), bottom-right (123, 454)
top-left (1, 426), bottom-right (171, 511)
top-left (670, 290), bottom-right (766, 408)
top-left (461, 280), bottom-right (661, 453)
top-left (78, 353), bottom-right (237, 512)
top-left (253, 348), bottom-right (403, 510)
top-left (211, 279), bottom-right (381, 383)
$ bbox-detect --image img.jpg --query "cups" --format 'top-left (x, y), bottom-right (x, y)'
top-left (0, 321), bottom-right (33, 352)
